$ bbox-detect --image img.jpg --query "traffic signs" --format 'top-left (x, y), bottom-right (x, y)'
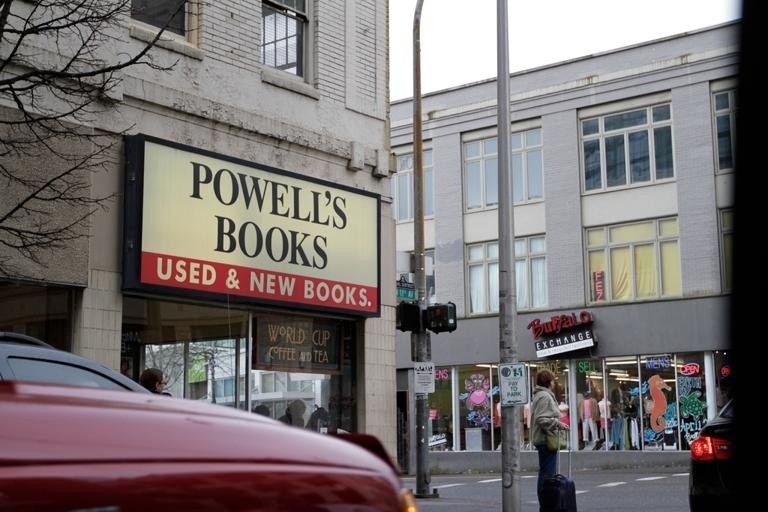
top-left (396, 287), bottom-right (415, 299)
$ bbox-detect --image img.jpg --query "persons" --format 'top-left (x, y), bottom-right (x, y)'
top-left (527, 367), bottom-right (571, 512)
top-left (514, 388), bottom-right (614, 446)
top-left (140, 367), bottom-right (173, 398)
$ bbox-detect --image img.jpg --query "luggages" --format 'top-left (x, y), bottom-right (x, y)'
top-left (540, 428), bottom-right (576, 512)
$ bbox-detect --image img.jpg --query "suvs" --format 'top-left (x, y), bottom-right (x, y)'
top-left (0, 328), bottom-right (153, 395)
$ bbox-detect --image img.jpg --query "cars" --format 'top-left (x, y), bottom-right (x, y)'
top-left (689, 395), bottom-right (733, 512)
top-left (0, 378), bottom-right (421, 511)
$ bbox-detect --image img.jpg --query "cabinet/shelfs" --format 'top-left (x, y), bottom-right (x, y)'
top-left (464, 427), bottom-right (490, 451)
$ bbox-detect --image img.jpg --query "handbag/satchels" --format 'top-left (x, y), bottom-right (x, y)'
top-left (545, 429), bottom-right (566, 450)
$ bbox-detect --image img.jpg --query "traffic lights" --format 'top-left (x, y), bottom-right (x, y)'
top-left (397, 302), bottom-right (423, 335)
top-left (426, 303), bottom-right (457, 333)
